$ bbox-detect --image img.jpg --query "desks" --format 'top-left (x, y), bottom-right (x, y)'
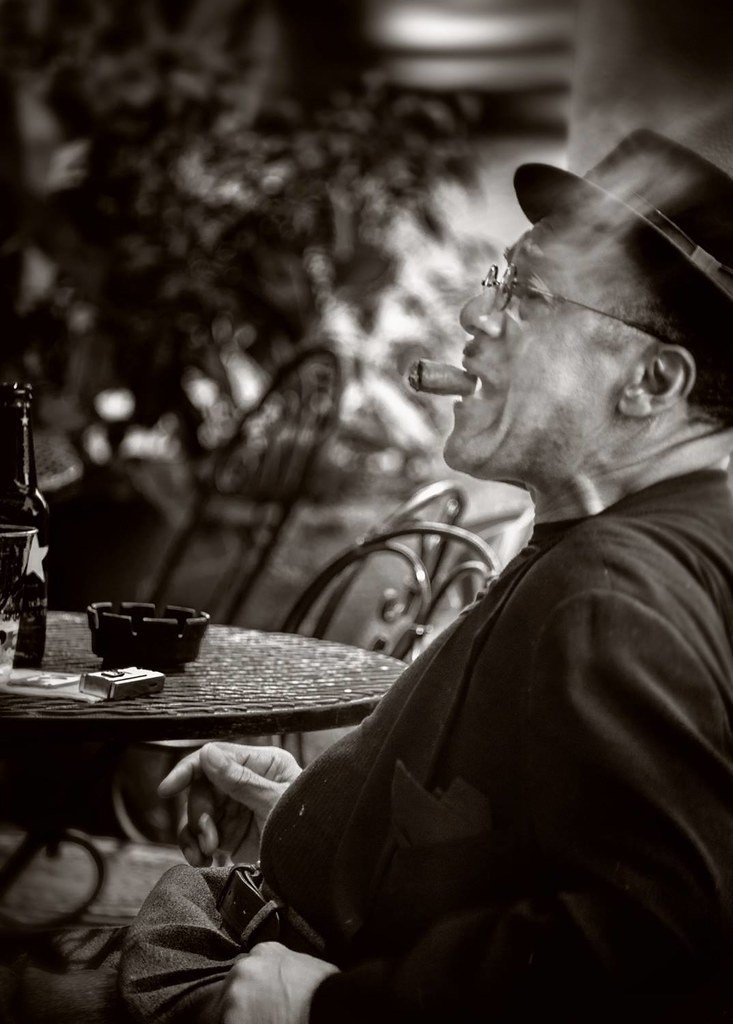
top-left (0, 611), bottom-right (412, 841)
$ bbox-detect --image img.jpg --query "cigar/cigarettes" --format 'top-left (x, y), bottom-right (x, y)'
top-left (408, 358), bottom-right (479, 396)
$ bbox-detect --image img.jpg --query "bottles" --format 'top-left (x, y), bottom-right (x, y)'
top-left (0, 382), bottom-right (54, 668)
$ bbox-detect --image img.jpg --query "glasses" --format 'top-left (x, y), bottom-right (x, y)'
top-left (482, 265), bottom-right (674, 345)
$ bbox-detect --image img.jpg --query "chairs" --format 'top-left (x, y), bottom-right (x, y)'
top-left (142, 342), bottom-right (343, 626)
top-left (256, 521), bottom-right (502, 748)
top-left (312, 480), bottom-right (467, 659)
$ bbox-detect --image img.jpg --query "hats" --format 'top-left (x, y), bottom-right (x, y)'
top-left (513, 128), bottom-right (733, 301)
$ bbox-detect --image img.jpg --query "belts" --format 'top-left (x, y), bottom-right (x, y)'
top-left (216, 862), bottom-right (318, 957)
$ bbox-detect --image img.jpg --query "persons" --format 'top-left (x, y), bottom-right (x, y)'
top-left (1, 124), bottom-right (733, 1024)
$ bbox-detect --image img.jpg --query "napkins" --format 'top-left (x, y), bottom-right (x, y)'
top-left (0, 668), bottom-right (104, 704)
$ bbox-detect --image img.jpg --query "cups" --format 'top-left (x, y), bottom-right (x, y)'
top-left (0, 523), bottom-right (39, 686)
